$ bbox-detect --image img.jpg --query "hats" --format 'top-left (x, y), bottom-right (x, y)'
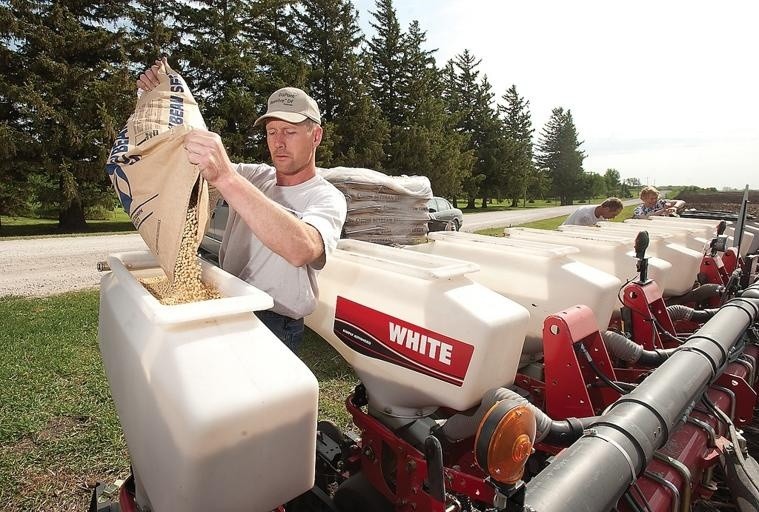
top-left (253, 86), bottom-right (322, 128)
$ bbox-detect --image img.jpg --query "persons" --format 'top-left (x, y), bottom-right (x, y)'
top-left (561, 197), bottom-right (623, 225)
top-left (633, 187), bottom-right (686, 219)
top-left (136, 57), bottom-right (347, 358)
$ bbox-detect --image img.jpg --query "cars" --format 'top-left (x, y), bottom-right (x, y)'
top-left (427, 196), bottom-right (464, 235)
